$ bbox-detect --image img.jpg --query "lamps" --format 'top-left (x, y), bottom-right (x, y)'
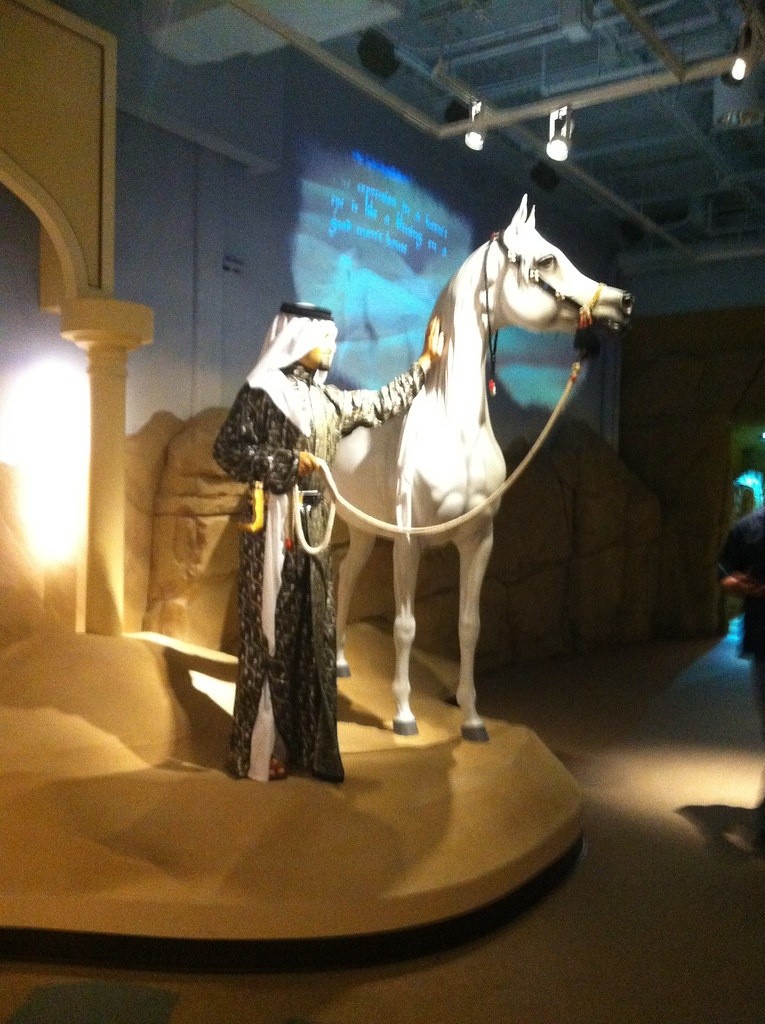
top-left (731, 55), bottom-right (750, 82)
top-left (464, 98), bottom-right (486, 153)
top-left (545, 103), bottom-right (579, 162)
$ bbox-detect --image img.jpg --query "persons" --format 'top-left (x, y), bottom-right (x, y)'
top-left (214, 303), bottom-right (445, 788)
top-left (717, 505), bottom-right (764, 847)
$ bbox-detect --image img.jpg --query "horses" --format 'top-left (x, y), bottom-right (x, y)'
top-left (323, 192), bottom-right (638, 744)
